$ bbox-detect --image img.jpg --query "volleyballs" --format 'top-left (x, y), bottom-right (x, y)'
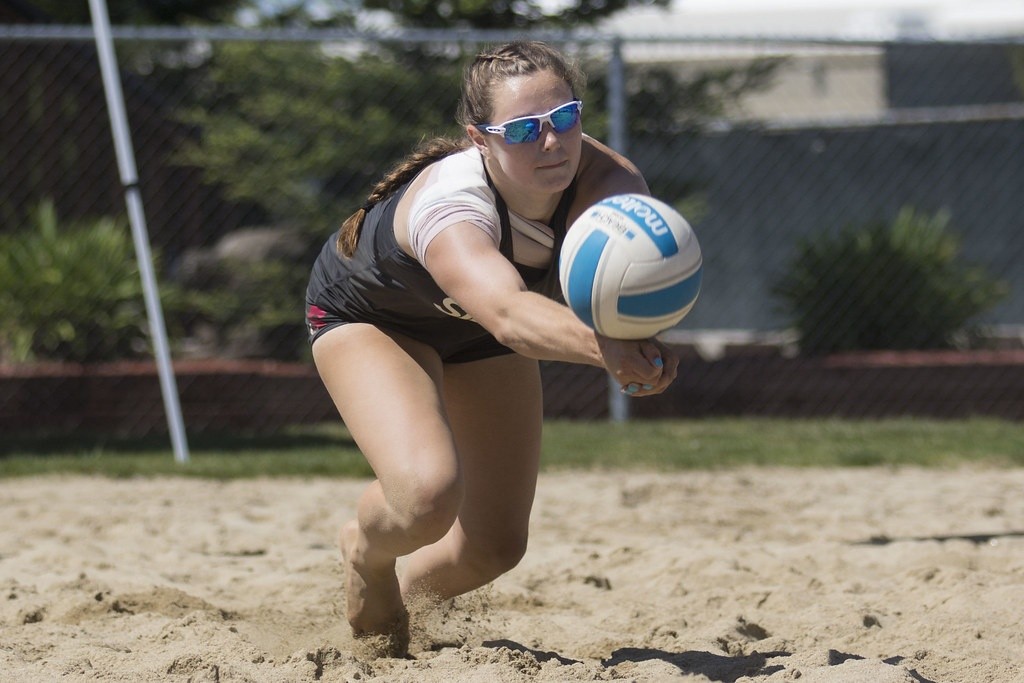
top-left (559, 194), bottom-right (702, 339)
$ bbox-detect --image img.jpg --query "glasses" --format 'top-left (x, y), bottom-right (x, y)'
top-left (473, 100), bottom-right (584, 145)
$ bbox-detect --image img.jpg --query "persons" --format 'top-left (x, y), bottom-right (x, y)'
top-left (303, 36), bottom-right (683, 659)
